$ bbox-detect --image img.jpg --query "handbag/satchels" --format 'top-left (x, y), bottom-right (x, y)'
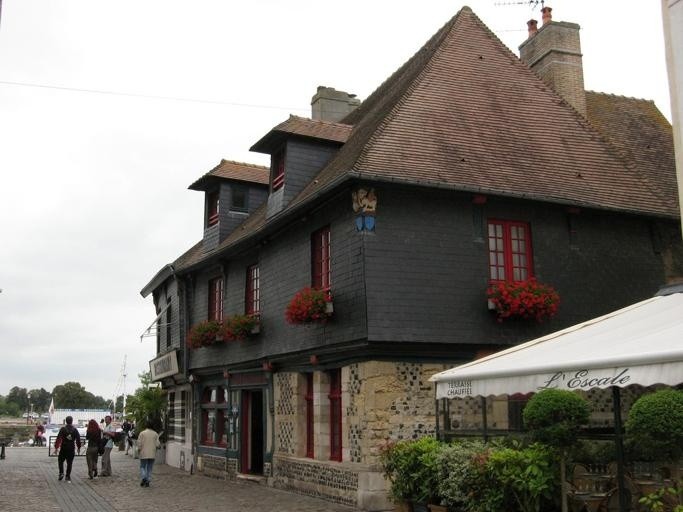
top-left (98, 437), bottom-right (108, 455)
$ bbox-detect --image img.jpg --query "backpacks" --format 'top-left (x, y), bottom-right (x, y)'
top-left (62, 426), bottom-right (75, 448)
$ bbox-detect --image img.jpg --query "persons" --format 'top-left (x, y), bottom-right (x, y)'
top-left (136, 423), bottom-right (161, 486)
top-left (121, 419), bottom-right (135, 455)
top-left (54, 416), bottom-right (81, 480)
top-left (30, 423), bottom-right (44, 447)
top-left (98, 419), bottom-right (106, 438)
top-left (98, 416), bottom-right (116, 477)
top-left (86, 419), bottom-right (101, 479)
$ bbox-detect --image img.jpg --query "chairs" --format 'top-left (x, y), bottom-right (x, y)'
top-left (553, 460), bottom-right (679, 512)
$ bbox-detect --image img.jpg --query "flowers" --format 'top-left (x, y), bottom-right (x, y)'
top-left (489, 278), bottom-right (560, 322)
top-left (186, 287), bottom-right (332, 350)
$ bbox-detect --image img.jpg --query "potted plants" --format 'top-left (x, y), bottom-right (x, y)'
top-left (379, 434), bottom-right (453, 512)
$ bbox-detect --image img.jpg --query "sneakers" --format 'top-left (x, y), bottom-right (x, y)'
top-left (141, 478), bottom-right (149, 487)
top-left (59, 474), bottom-right (71, 481)
top-left (89, 470), bottom-right (97, 479)
